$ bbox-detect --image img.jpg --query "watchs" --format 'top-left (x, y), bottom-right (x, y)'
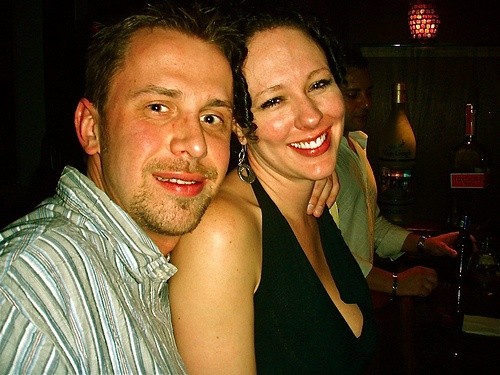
top-left (417, 234), bottom-right (430, 251)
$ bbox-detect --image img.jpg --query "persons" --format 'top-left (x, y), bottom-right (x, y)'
top-left (275, 43), bottom-right (481, 375)
top-left (0, 0), bottom-right (256, 372)
top-left (166, 6), bottom-right (382, 375)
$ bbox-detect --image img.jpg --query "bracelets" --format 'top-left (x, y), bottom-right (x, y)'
top-left (392, 271), bottom-right (399, 300)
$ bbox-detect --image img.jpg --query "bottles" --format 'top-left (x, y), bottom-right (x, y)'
top-left (448, 101), bottom-right (489, 231)
top-left (453, 218), bottom-right (473, 277)
top-left (477, 236), bottom-right (496, 297)
top-left (377, 81), bottom-right (417, 207)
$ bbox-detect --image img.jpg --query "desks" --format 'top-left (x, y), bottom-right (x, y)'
top-left (372, 222), bottom-right (500, 375)
